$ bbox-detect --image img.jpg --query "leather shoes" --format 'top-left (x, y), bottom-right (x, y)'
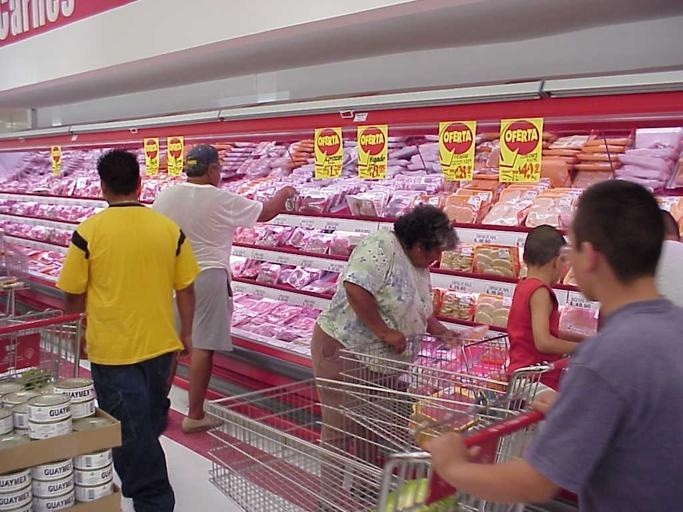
top-left (181, 411), bottom-right (224, 435)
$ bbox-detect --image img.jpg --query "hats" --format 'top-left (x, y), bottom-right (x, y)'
top-left (185, 144), bottom-right (220, 165)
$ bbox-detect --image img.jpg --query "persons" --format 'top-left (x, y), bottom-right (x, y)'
top-left (54, 149), bottom-right (202, 511)
top-left (595, 209), bottom-right (681, 335)
top-left (149, 142), bottom-right (300, 434)
top-left (503, 223), bottom-right (591, 408)
top-left (419, 180), bottom-right (682, 512)
top-left (307, 203), bottom-right (463, 512)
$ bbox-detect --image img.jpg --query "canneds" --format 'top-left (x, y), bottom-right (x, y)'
top-left (0, 378), bottom-right (115, 512)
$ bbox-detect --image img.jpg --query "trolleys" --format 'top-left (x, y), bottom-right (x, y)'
top-left (198, 330), bottom-right (574, 512)
top-left (0, 229), bottom-right (88, 382)
